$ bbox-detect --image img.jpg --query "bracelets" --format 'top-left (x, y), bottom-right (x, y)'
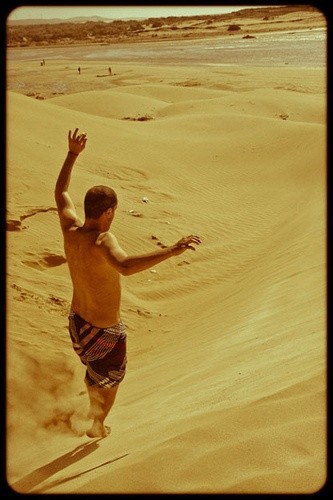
top-left (68, 151), bottom-right (78, 157)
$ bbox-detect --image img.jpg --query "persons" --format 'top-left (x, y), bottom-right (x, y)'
top-left (52, 127), bottom-right (203, 438)
